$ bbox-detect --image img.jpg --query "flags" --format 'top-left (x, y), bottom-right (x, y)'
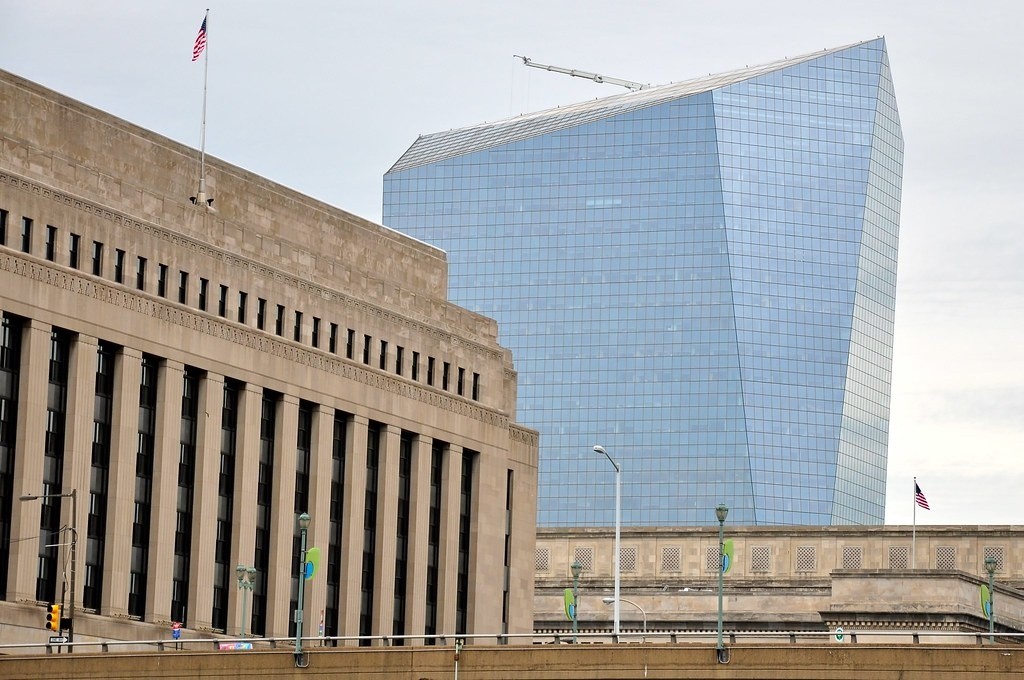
top-left (916, 483), bottom-right (930, 510)
top-left (192, 15), bottom-right (207, 62)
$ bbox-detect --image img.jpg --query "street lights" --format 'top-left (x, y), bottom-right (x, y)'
top-left (594, 445), bottom-right (622, 644)
top-left (19, 488), bottom-right (76, 654)
top-left (716, 502), bottom-right (728, 650)
top-left (294, 511), bottom-right (312, 655)
top-left (570, 560), bottom-right (583, 642)
top-left (984, 555), bottom-right (998, 647)
top-left (234, 563), bottom-right (257, 639)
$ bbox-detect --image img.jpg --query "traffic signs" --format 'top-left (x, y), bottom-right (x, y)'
top-left (48, 636), bottom-right (69, 644)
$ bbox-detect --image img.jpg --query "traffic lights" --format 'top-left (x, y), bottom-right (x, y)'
top-left (45, 604), bottom-right (59, 633)
